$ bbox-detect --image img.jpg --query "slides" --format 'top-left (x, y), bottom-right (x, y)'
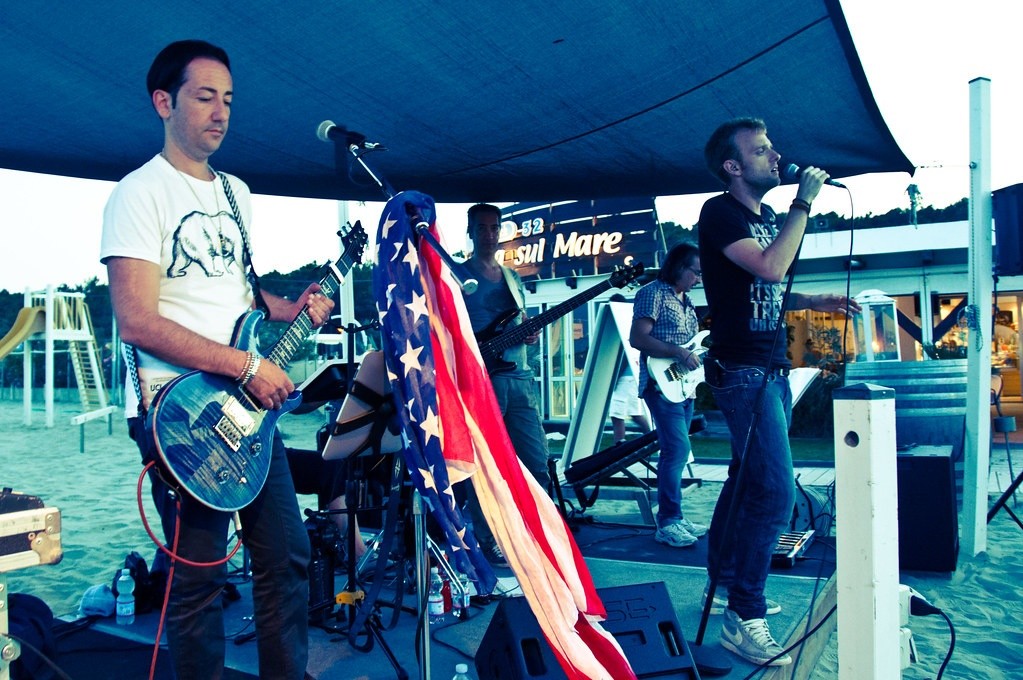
top-left (0, 306), bottom-right (46, 362)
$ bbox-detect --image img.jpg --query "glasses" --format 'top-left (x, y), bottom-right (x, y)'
top-left (689, 268), bottom-right (703, 278)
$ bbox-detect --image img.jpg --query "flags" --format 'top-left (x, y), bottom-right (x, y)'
top-left (370, 195), bottom-right (642, 680)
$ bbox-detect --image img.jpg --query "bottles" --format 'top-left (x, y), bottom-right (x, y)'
top-left (429, 567), bottom-right (444, 624)
top-left (116, 568), bottom-right (136, 627)
top-left (452, 664), bottom-right (470, 680)
top-left (453, 575), bottom-right (470, 618)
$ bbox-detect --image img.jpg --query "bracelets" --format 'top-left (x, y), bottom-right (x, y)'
top-left (235, 350), bottom-right (261, 386)
top-left (789, 199), bottom-right (811, 214)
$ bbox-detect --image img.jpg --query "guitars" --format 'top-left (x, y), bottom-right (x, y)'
top-left (473, 260), bottom-right (646, 379)
top-left (145, 218), bottom-right (369, 517)
top-left (647, 328), bottom-right (711, 405)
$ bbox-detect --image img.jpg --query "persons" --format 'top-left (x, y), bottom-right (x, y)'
top-left (455, 202), bottom-right (554, 568)
top-left (698, 116), bottom-right (832, 665)
top-left (628, 243), bottom-right (708, 548)
top-left (99, 38), bottom-right (334, 680)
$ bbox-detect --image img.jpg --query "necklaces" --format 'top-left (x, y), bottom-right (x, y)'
top-left (165, 158), bottom-right (225, 243)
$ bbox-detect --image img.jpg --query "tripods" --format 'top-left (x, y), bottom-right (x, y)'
top-left (232, 480), bottom-right (410, 680)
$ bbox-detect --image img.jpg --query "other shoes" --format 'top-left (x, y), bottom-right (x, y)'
top-left (355, 549), bottom-right (394, 571)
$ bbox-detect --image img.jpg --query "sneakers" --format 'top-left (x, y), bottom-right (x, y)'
top-left (701, 576), bottom-right (781, 614)
top-left (654, 518), bottom-right (707, 547)
top-left (719, 605), bottom-right (792, 666)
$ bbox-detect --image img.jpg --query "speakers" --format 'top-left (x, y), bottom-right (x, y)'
top-left (897, 443), bottom-right (961, 573)
top-left (474, 581), bottom-right (701, 680)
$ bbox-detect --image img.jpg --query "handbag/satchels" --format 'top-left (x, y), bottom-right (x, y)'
top-left (112, 548), bottom-right (153, 614)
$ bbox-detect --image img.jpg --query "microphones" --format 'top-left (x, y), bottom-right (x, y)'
top-left (314, 117), bottom-right (389, 156)
top-left (783, 160), bottom-right (849, 190)
